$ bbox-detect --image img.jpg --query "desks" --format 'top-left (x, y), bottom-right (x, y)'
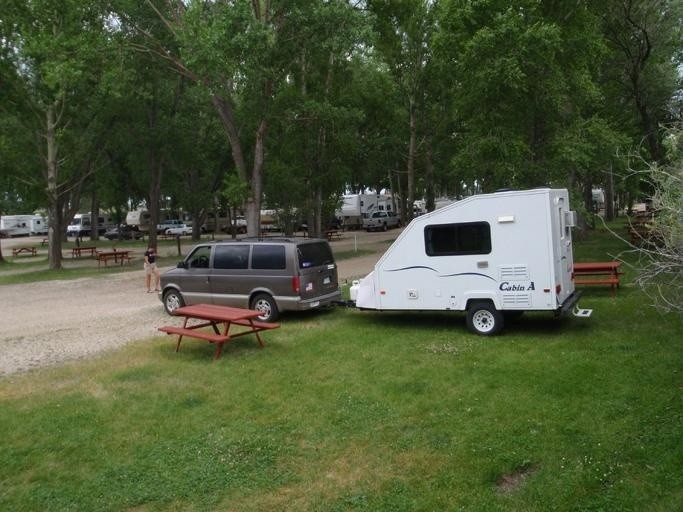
top-left (172, 304), bottom-right (263, 359)
top-left (40, 237), bottom-right (48, 242)
top-left (574, 262), bottom-right (624, 299)
top-left (326, 231), bottom-right (340, 240)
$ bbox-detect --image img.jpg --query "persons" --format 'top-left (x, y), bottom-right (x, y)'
top-left (143, 244), bottom-right (162, 293)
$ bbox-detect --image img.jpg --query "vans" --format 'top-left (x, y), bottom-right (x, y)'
top-left (158, 239), bottom-right (342, 324)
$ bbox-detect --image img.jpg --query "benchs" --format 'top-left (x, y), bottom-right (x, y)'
top-left (12, 242), bottom-right (135, 267)
top-left (329, 235), bottom-right (341, 236)
top-left (574, 270), bottom-right (625, 284)
top-left (231, 319), bottom-right (280, 329)
top-left (158, 326), bottom-right (230, 342)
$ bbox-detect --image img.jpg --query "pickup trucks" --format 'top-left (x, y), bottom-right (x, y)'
top-left (362, 210), bottom-right (402, 232)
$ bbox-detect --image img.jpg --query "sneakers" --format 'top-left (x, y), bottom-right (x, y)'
top-left (147, 290), bottom-right (153, 293)
top-left (155, 289), bottom-right (161, 292)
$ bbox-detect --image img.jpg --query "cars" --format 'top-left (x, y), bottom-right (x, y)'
top-left (104, 228), bottom-right (144, 240)
top-left (165, 224), bottom-right (193, 236)
